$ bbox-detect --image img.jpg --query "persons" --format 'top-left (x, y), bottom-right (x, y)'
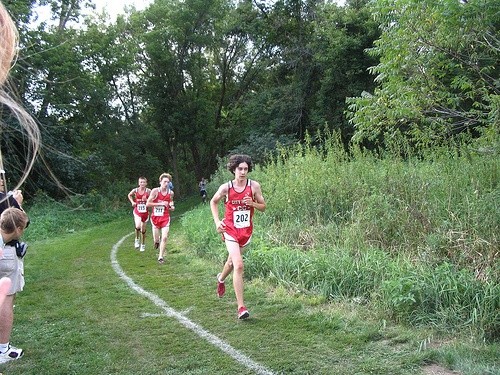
top-left (210, 156), bottom-right (265, 319)
top-left (0, 165), bottom-right (28, 364)
top-left (128, 172), bottom-right (175, 261)
top-left (0, 1), bottom-right (42, 201)
top-left (199, 178), bottom-right (209, 205)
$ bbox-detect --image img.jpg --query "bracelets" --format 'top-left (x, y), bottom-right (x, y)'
top-left (170, 204), bottom-right (174, 207)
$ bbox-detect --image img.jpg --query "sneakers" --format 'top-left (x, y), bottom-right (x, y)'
top-left (135, 237), bottom-right (140, 248)
top-left (139, 244), bottom-right (145, 252)
top-left (217, 273), bottom-right (226, 298)
top-left (237, 306), bottom-right (249, 320)
top-left (0, 345), bottom-right (24, 364)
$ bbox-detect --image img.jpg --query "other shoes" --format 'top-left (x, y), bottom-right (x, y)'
top-left (154, 243), bottom-right (159, 249)
top-left (159, 257), bottom-right (164, 264)
